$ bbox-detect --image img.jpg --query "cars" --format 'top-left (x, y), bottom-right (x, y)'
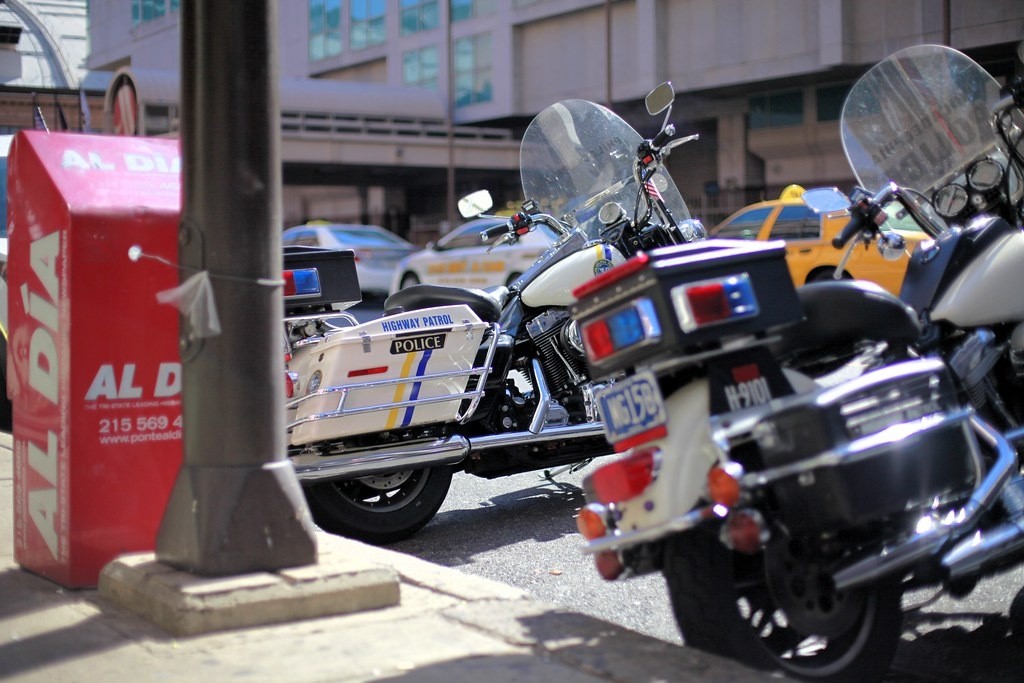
top-left (707, 184), bottom-right (931, 297)
top-left (389, 219), bottom-right (560, 297)
top-left (283, 219), bottom-right (420, 307)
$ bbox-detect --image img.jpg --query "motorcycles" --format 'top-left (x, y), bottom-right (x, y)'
top-left (282, 80), bottom-right (706, 546)
top-left (572, 43), bottom-right (1024, 682)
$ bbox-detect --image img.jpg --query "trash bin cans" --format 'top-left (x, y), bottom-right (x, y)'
top-left (7, 130), bottom-right (186, 589)
